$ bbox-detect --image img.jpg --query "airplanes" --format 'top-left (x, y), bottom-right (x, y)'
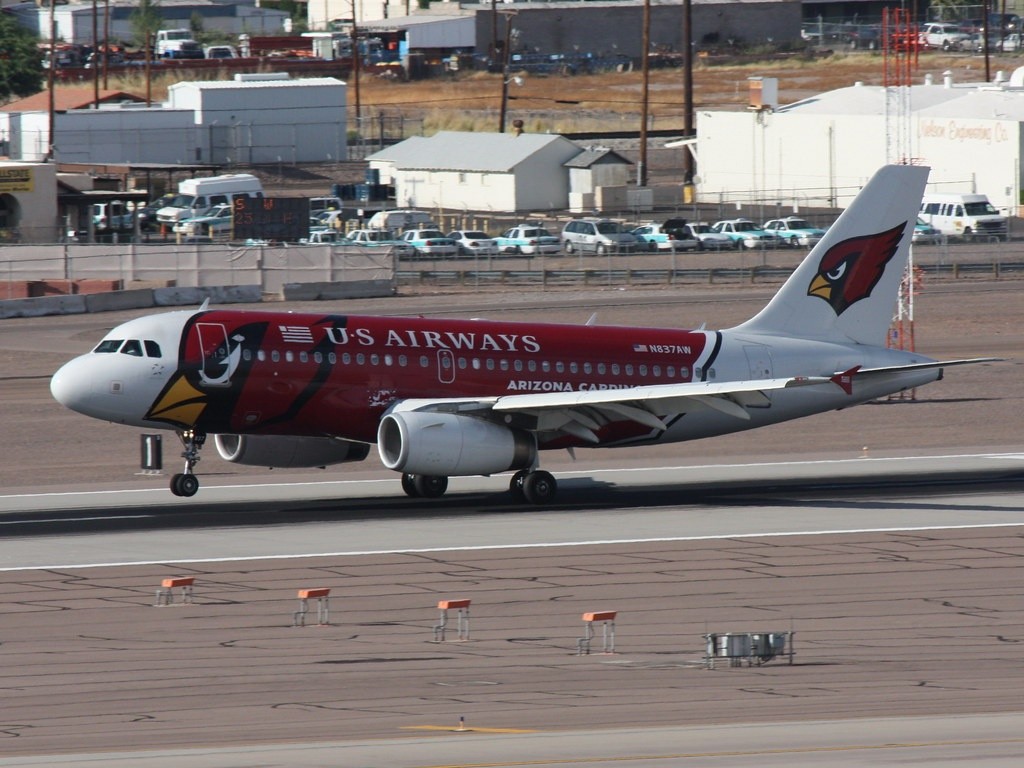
top-left (49, 164), bottom-right (1012, 512)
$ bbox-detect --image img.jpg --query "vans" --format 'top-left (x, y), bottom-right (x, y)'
top-left (918, 193), bottom-right (1009, 242)
top-left (560, 218), bottom-right (639, 256)
top-left (154, 173), bottom-right (266, 231)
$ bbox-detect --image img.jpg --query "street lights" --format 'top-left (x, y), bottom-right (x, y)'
top-left (497, 74), bottom-right (526, 133)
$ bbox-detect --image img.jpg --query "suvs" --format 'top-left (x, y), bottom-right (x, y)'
top-left (153, 28), bottom-right (204, 59)
top-left (93, 202), bottom-right (134, 234)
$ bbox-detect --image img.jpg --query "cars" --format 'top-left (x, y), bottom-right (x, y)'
top-left (397, 227), bottom-right (459, 256)
top-left (345, 227), bottom-right (416, 259)
top-left (173, 201), bottom-right (232, 237)
top-left (42, 44), bottom-right (126, 73)
top-left (684, 222), bottom-right (736, 251)
top-left (709, 218), bottom-right (782, 249)
top-left (245, 198), bottom-right (433, 246)
top-left (846, 11), bottom-right (1024, 54)
top-left (445, 229), bottom-right (500, 256)
top-left (762, 214), bottom-right (827, 251)
top-left (629, 218), bottom-right (699, 253)
top-left (204, 46), bottom-right (239, 59)
top-left (492, 223), bottom-right (562, 255)
top-left (136, 192), bottom-right (179, 228)
top-left (910, 217), bottom-right (942, 245)
